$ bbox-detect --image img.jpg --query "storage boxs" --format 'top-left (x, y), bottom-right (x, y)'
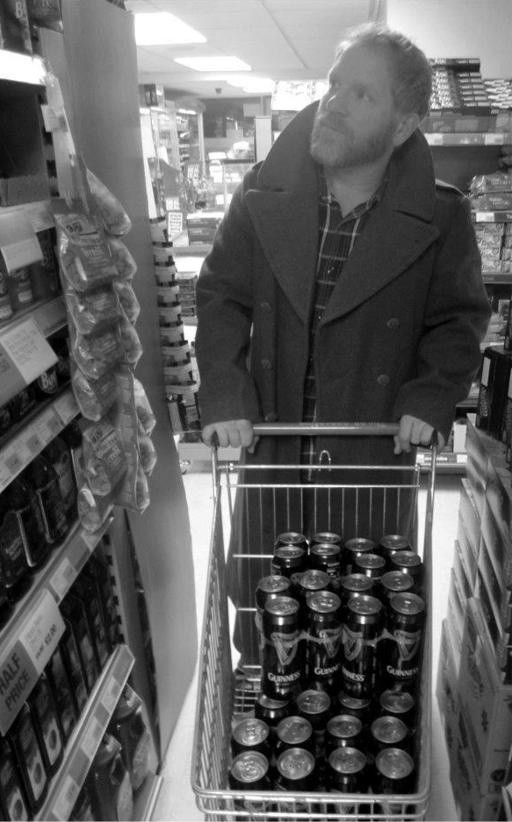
top-left (433, 428), bottom-right (512, 822)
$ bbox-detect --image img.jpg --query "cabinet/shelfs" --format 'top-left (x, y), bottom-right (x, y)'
top-left (415, 130), bottom-right (512, 476)
top-left (2, 43), bottom-right (169, 822)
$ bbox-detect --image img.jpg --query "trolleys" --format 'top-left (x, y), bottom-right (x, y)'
top-left (183, 413), bottom-right (443, 821)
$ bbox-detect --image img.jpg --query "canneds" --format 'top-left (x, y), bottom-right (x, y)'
top-left (228, 532), bottom-right (426, 822)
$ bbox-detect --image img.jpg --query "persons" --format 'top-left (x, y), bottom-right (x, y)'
top-left (194, 23), bottom-right (492, 720)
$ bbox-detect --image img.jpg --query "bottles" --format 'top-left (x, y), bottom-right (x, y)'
top-left (1, 227), bottom-right (162, 821)
